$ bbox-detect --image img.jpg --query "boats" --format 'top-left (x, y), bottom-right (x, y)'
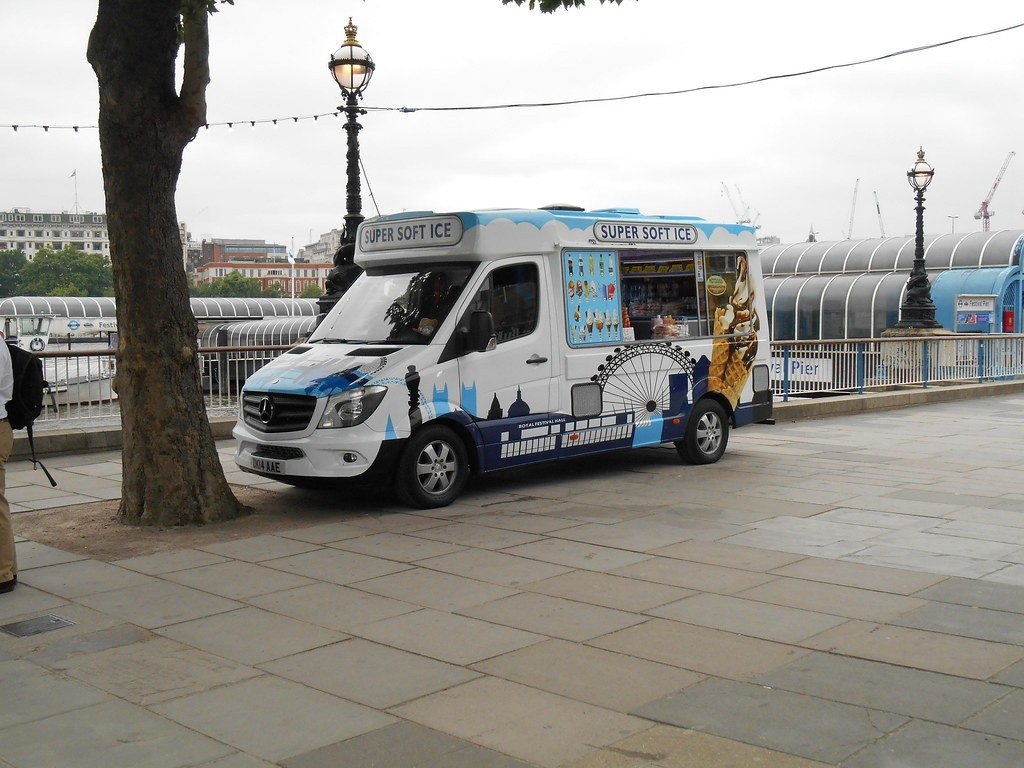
top-left (0, 314), bottom-right (118, 406)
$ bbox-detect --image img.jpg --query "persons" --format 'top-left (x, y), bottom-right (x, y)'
top-left (420, 270), bottom-right (530, 344)
top-left (0, 335), bottom-right (18, 594)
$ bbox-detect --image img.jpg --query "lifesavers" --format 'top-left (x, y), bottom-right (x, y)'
top-left (30, 337), bottom-right (45, 351)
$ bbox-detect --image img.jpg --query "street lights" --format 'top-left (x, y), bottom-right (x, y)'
top-left (315, 15), bottom-right (374, 329)
top-left (893, 147), bottom-right (942, 329)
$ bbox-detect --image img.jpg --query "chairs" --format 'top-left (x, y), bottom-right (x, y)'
top-left (490, 269), bottom-right (526, 334)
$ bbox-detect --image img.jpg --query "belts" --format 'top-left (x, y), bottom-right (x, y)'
top-left (0, 418), bottom-right (8, 423)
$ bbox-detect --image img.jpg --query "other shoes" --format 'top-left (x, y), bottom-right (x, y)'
top-left (0, 575), bottom-right (17, 594)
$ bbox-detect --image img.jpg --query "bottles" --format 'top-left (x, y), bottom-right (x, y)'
top-left (655, 315), bottom-right (672, 326)
top-left (569, 253), bottom-right (613, 277)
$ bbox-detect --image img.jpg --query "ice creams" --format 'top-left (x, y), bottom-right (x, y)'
top-left (586, 308), bottom-right (618, 333)
top-left (706, 256), bottom-right (761, 411)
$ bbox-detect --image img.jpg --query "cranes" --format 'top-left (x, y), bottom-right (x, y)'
top-left (720, 150), bottom-right (1017, 233)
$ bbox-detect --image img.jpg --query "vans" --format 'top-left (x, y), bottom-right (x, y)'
top-left (230, 198), bottom-right (774, 509)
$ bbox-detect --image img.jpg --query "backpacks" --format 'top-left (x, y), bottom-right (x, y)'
top-left (5, 343), bottom-right (43, 430)
